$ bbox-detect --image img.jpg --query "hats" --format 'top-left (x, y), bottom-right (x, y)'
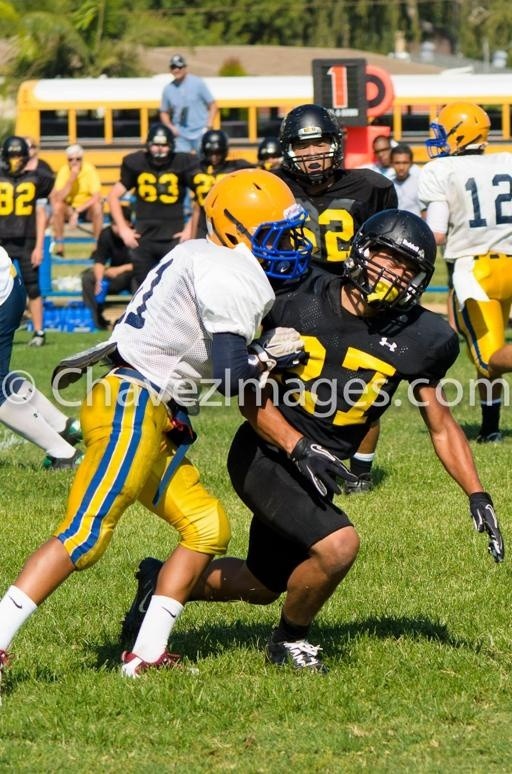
top-left (170, 54), bottom-right (186, 68)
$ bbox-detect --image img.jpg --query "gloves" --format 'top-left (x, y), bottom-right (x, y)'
top-left (469, 492), bottom-right (504, 563)
top-left (248, 326), bottom-right (309, 372)
top-left (290, 437), bottom-right (359, 498)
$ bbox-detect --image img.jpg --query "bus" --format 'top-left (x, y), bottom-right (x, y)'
top-left (9, 60), bottom-right (512, 224)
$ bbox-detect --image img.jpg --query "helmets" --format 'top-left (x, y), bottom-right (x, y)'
top-left (343, 209), bottom-right (437, 281)
top-left (202, 129), bottom-right (227, 155)
top-left (429, 101), bottom-right (492, 155)
top-left (147, 125), bottom-right (174, 148)
top-left (258, 137), bottom-right (284, 160)
top-left (279, 104), bottom-right (344, 166)
top-left (2, 136), bottom-right (28, 165)
top-left (203, 168), bottom-right (311, 252)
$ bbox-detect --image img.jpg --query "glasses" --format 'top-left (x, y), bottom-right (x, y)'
top-left (68, 157), bottom-right (80, 161)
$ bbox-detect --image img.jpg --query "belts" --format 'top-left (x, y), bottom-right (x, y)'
top-left (111, 368), bottom-right (188, 418)
top-left (474, 254), bottom-right (512, 260)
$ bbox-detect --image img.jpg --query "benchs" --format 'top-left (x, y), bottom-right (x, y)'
top-left (38, 200), bottom-right (147, 301)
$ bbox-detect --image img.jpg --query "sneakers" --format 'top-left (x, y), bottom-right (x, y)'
top-left (28, 331), bottom-right (45, 346)
top-left (59, 417), bottom-right (83, 445)
top-left (44, 449), bottom-right (84, 472)
top-left (118, 557), bottom-right (163, 650)
top-left (265, 626), bottom-right (329, 676)
top-left (121, 649), bottom-right (200, 681)
top-left (345, 472), bottom-right (374, 494)
